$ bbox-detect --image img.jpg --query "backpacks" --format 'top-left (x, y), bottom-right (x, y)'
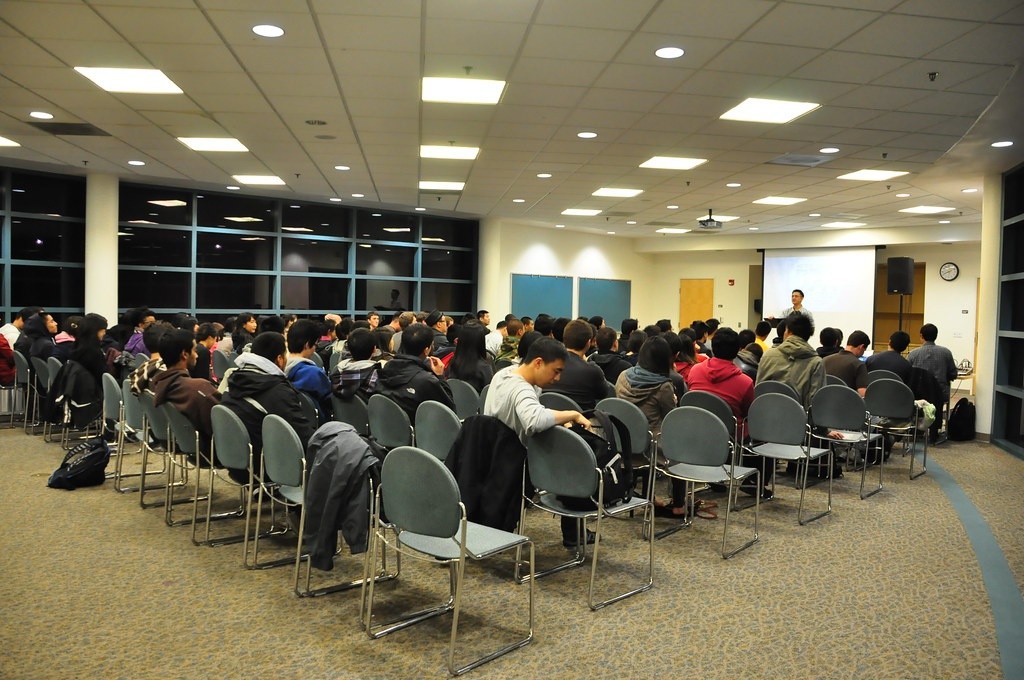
top-left (560, 408), bottom-right (630, 511)
top-left (46, 436), bottom-right (111, 491)
top-left (806, 426), bottom-right (844, 479)
top-left (950, 400), bottom-right (977, 443)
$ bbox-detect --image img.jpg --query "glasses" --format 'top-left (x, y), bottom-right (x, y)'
top-left (792, 295), bottom-right (804, 298)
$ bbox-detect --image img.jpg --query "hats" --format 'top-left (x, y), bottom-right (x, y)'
top-left (424, 309), bottom-right (444, 325)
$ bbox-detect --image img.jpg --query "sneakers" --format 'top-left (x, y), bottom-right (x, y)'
top-left (560, 532), bottom-right (602, 545)
top-left (739, 479), bottom-right (772, 497)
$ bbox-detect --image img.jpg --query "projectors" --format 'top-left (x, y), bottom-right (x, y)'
top-left (699, 221), bottom-right (722, 228)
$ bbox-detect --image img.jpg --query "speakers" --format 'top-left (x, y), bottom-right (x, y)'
top-left (887, 257), bottom-right (914, 296)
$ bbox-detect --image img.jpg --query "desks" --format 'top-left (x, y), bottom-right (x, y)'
top-left (956, 374), bottom-right (974, 379)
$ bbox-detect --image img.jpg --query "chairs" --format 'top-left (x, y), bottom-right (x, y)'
top-left (0, 350), bottom-right (947, 676)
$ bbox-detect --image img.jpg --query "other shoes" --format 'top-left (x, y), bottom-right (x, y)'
top-left (663, 506), bottom-right (688, 518)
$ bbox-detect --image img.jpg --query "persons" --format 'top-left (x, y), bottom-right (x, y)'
top-left (0, 310), bottom-right (958, 547)
top-left (389, 289), bottom-right (402, 310)
top-left (768, 290), bottom-right (814, 336)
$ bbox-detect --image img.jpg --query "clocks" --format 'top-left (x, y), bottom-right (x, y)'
top-left (940, 262), bottom-right (959, 281)
